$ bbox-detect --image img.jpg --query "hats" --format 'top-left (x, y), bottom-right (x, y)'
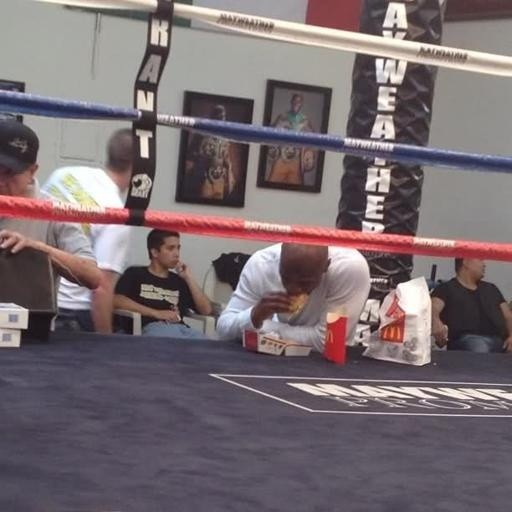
top-left (0, 117), bottom-right (40, 174)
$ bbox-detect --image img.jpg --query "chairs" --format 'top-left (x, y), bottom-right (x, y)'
top-left (113, 252), bottom-right (256, 340)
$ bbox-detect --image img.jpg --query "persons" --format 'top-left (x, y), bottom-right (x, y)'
top-left (39, 128), bottom-right (133, 331)
top-left (0, 118), bottom-right (101, 333)
top-left (431, 257), bottom-right (512, 355)
top-left (215, 242), bottom-right (371, 355)
top-left (113, 229), bottom-right (212, 338)
top-left (185, 105), bottom-right (234, 200)
top-left (268, 95), bottom-right (315, 185)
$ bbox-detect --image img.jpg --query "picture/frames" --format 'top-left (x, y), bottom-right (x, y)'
top-left (174, 90), bottom-right (255, 209)
top-left (254, 78), bottom-right (333, 194)
top-left (0, 77), bottom-right (28, 128)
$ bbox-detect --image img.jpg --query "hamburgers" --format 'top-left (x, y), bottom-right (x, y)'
top-left (290, 293), bottom-right (309, 315)
